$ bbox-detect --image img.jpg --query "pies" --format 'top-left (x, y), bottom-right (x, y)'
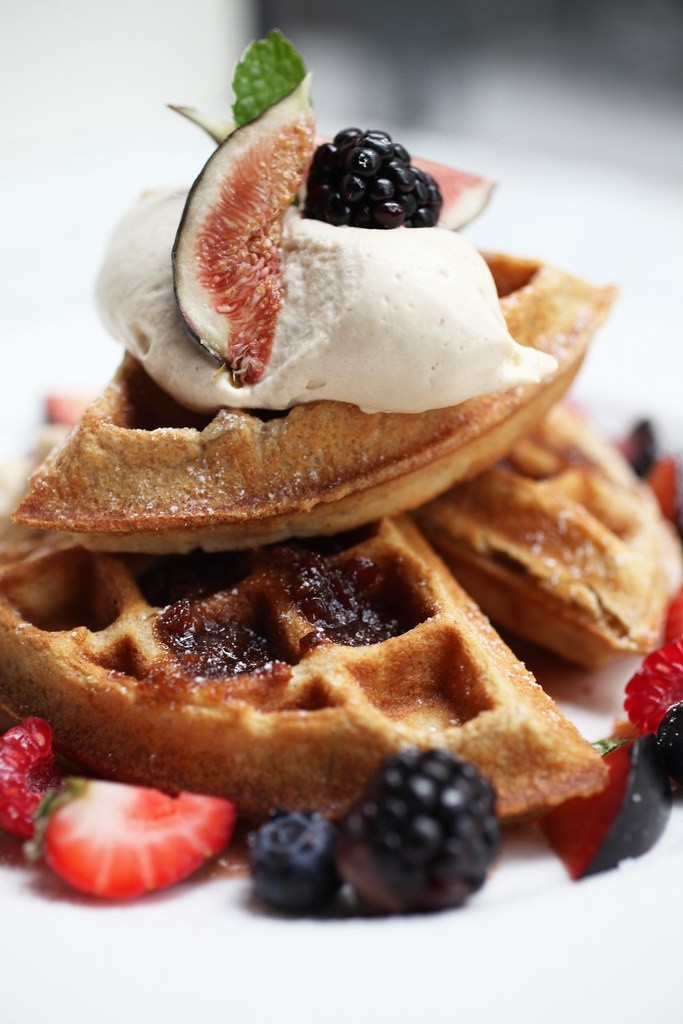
top-left (0, 254), bottom-right (672, 829)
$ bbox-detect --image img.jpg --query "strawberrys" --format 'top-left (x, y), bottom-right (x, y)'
top-left (28, 777), bottom-right (238, 903)
